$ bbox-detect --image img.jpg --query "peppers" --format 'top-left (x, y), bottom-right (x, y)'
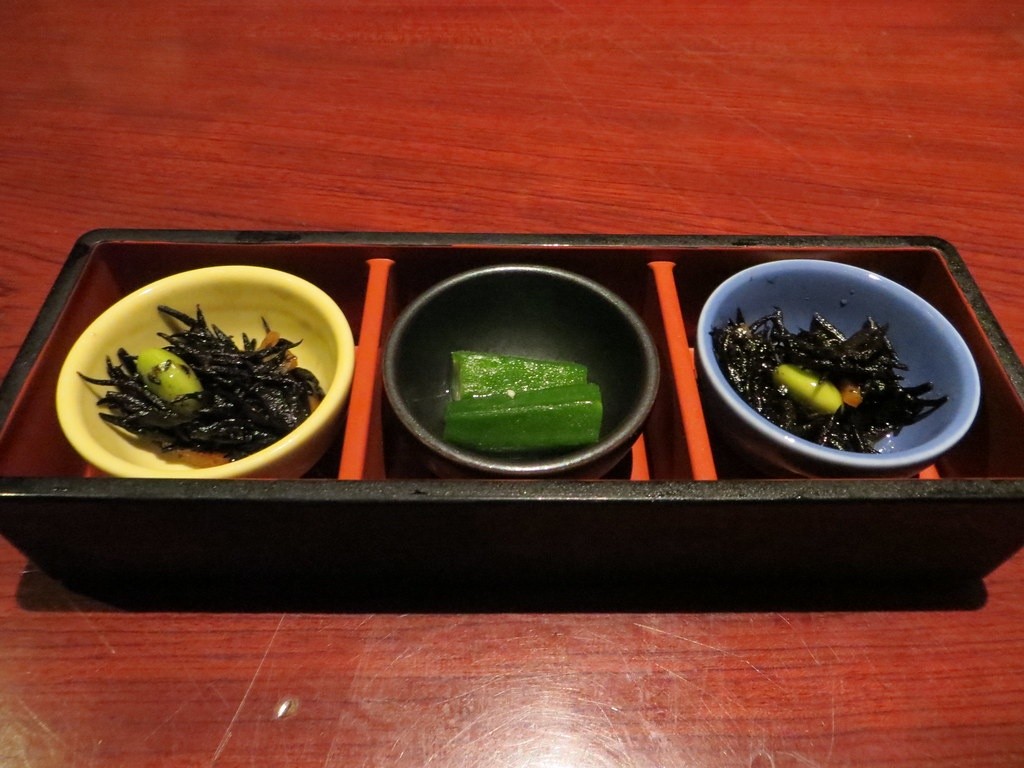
top-left (124, 349), bottom-right (203, 415)
top-left (771, 364), bottom-right (844, 414)
top-left (440, 350), bottom-right (601, 450)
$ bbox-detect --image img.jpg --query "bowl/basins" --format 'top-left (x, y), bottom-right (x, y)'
top-left (56, 264), bottom-right (354, 478)
top-left (382, 262), bottom-right (660, 476)
top-left (698, 258), bottom-right (981, 466)
